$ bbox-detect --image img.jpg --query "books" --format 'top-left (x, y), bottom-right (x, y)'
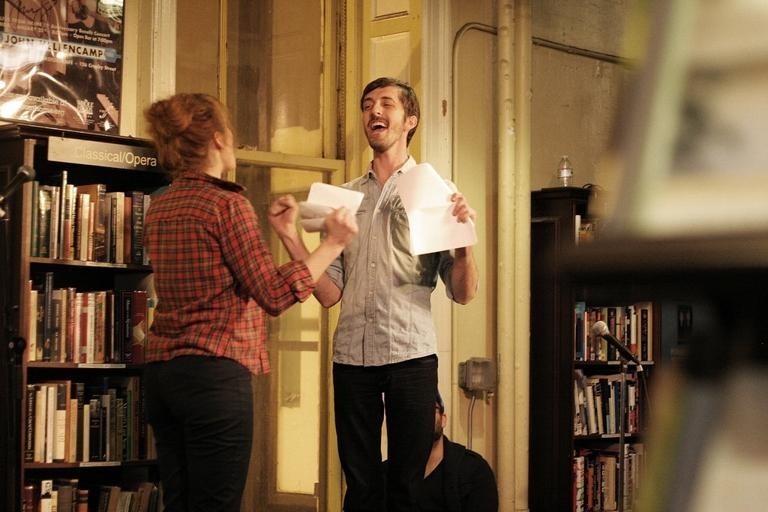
top-left (573, 441), bottom-right (653, 511)
top-left (27, 378), bottom-right (150, 463)
top-left (32, 170), bottom-right (155, 265)
top-left (574, 369), bottom-right (656, 435)
top-left (575, 304), bottom-right (658, 364)
top-left (20, 476), bottom-right (165, 512)
top-left (28, 272), bottom-right (155, 364)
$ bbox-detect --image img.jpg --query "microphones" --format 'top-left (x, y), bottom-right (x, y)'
top-left (592, 320), bottom-right (639, 365)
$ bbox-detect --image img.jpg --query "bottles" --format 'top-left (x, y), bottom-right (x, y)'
top-left (557, 155), bottom-right (574, 188)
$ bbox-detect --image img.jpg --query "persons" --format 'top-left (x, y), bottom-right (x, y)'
top-left (142, 93), bottom-right (359, 512)
top-left (268, 77), bottom-right (479, 511)
top-left (342, 386), bottom-right (499, 512)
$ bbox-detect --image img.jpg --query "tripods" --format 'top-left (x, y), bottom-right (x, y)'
top-left (617, 356), bottom-right (626, 512)
